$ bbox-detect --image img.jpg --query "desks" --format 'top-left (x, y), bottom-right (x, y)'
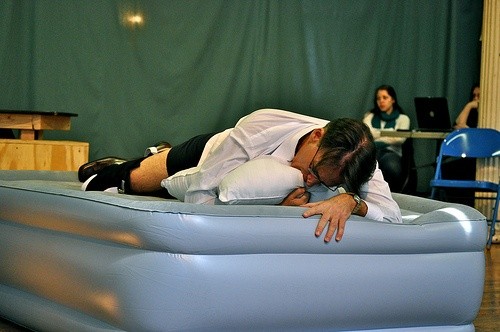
top-left (381, 130), bottom-right (450, 138)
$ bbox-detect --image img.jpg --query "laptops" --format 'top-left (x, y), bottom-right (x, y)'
top-left (414, 97), bottom-right (452, 132)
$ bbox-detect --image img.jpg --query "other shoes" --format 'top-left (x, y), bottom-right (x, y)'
top-left (81, 164), bottom-right (118, 191)
top-left (78, 156), bottom-right (126, 183)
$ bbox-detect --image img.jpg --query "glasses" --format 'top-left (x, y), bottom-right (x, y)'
top-left (308, 143), bottom-right (340, 193)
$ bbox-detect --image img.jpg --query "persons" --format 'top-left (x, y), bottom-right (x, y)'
top-left (77, 107), bottom-right (403, 243)
top-left (361, 85), bottom-right (411, 194)
top-left (454, 83), bottom-right (480, 131)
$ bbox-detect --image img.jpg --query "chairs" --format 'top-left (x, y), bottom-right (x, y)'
top-left (390, 129), bottom-right (417, 193)
top-left (429, 128), bottom-right (500, 251)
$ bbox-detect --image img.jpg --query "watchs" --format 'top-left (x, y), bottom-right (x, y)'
top-left (345, 193), bottom-right (362, 215)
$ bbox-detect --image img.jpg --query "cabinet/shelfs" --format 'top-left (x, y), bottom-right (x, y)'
top-left (0, 110), bottom-right (90, 170)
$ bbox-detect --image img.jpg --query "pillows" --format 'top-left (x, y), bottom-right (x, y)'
top-left (160, 154), bottom-right (304, 204)
